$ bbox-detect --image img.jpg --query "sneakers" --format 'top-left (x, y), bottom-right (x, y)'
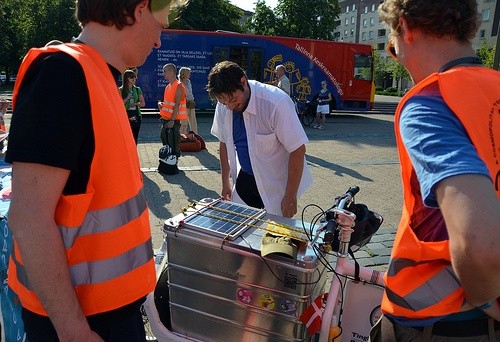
top-left (313, 124), bottom-right (319, 129)
top-left (318, 126), bottom-right (325, 130)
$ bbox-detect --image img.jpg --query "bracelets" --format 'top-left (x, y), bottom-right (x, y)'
top-left (171, 120), bottom-right (175, 122)
top-left (479, 299), bottom-right (497, 309)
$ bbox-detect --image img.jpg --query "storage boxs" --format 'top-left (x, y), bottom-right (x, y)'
top-left (159, 197), bottom-right (329, 342)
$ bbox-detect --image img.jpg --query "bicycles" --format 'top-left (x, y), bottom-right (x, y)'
top-left (293, 97), bottom-right (315, 126)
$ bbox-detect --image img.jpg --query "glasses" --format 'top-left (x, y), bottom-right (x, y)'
top-left (385, 24), bottom-right (400, 61)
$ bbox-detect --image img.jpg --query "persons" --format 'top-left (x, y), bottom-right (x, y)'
top-left (176, 67), bottom-right (198, 133)
top-left (5, 0), bottom-right (188, 342)
top-left (313, 80), bottom-right (332, 130)
top-left (377, 0), bottom-right (500, 342)
top-left (205, 62), bottom-right (313, 220)
top-left (275, 65), bottom-right (290, 96)
top-left (158, 63), bottom-right (186, 159)
top-left (118, 70), bottom-right (145, 145)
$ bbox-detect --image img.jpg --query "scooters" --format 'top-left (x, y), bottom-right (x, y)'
top-left (145, 185), bottom-right (389, 342)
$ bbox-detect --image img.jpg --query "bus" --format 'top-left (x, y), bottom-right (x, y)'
top-left (119, 29), bottom-right (376, 113)
